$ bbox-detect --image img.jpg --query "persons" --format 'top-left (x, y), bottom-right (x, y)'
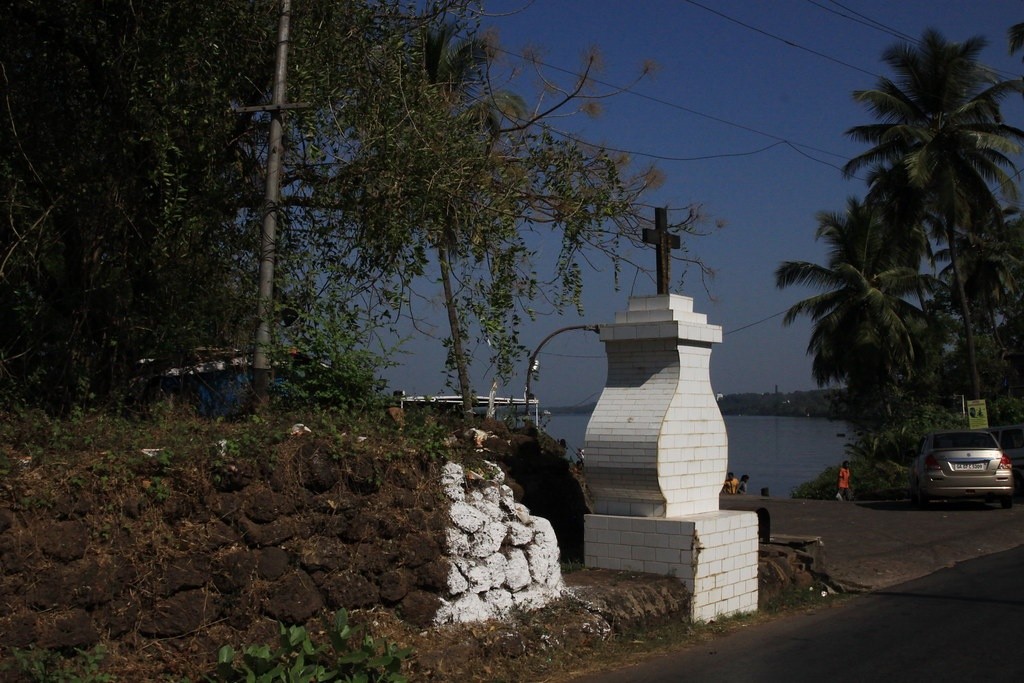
top-left (838, 461), bottom-right (852, 501)
top-left (737, 475), bottom-right (750, 496)
top-left (725, 473), bottom-right (740, 495)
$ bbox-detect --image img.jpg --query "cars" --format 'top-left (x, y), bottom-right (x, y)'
top-left (986, 426), bottom-right (1024, 471)
top-left (912, 430), bottom-right (1012, 511)
